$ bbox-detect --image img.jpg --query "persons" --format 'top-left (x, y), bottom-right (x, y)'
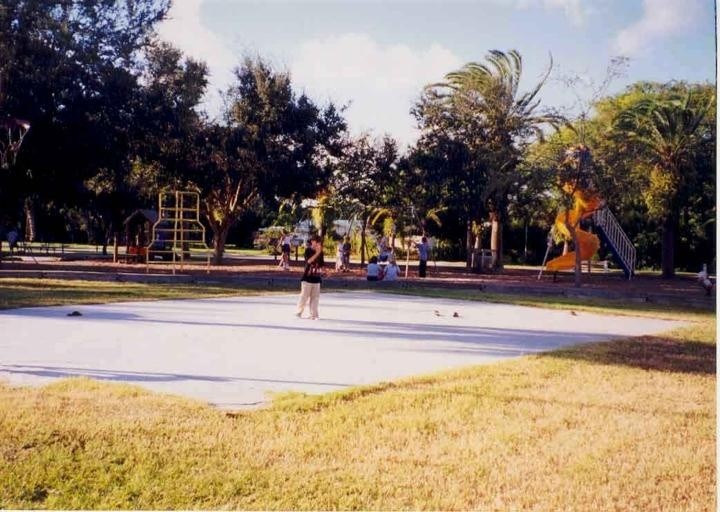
top-left (277, 228), bottom-right (292, 268)
top-left (7, 226), bottom-right (20, 253)
top-left (334, 237), bottom-right (345, 272)
top-left (341, 237), bottom-right (352, 272)
top-left (367, 256), bottom-right (384, 281)
top-left (280, 231), bottom-right (292, 270)
top-left (412, 237), bottom-right (430, 278)
top-left (295, 234), bottom-right (326, 321)
top-left (381, 256), bottom-right (402, 281)
top-left (375, 246), bottom-right (394, 263)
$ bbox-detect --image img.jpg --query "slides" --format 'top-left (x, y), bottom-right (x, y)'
top-left (547, 174), bottom-right (605, 271)
